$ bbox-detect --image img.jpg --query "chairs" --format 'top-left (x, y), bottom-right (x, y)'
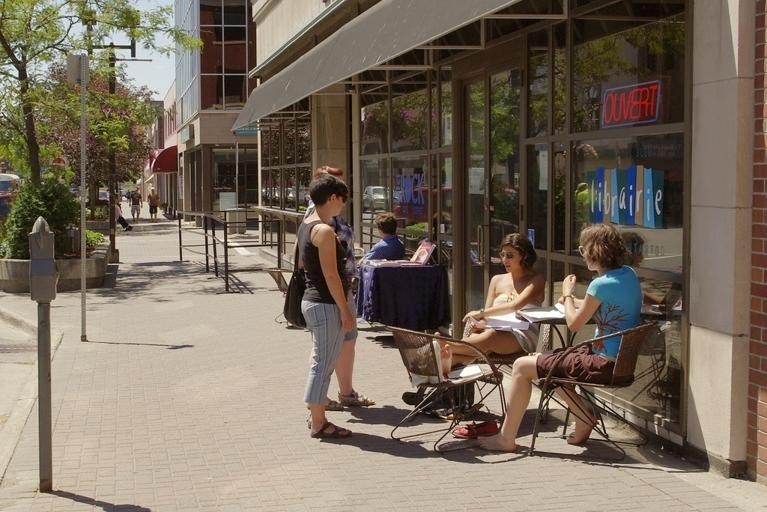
top-left (457, 351), bottom-right (534, 420)
top-left (281, 252), bottom-right (299, 269)
top-left (266, 265), bottom-right (301, 331)
top-left (350, 246), bottom-right (367, 268)
top-left (529, 320), bottom-right (663, 459)
top-left (379, 323), bottom-right (512, 456)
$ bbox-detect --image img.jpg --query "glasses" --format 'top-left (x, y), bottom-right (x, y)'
top-left (499, 251), bottom-right (515, 259)
top-left (579, 245), bottom-right (585, 256)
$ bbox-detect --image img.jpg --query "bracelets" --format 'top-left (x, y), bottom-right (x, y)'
top-left (563, 294), bottom-right (573, 302)
top-left (480, 308), bottom-right (484, 312)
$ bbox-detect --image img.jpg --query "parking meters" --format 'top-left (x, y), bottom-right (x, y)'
top-left (29, 211), bottom-right (61, 494)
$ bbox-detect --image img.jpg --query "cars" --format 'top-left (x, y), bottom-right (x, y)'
top-left (98, 191), bottom-right (108, 202)
top-left (262, 187), bottom-right (312, 208)
top-left (362, 185), bottom-right (386, 211)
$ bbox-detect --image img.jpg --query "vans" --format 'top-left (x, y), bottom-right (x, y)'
top-left (0, 173), bottom-right (20, 217)
top-left (391, 184), bottom-right (451, 233)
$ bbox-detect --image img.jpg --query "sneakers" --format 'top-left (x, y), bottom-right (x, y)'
top-left (402, 392), bottom-right (452, 409)
top-left (453, 419), bottom-right (499, 437)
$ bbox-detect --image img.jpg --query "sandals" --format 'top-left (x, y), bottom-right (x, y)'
top-left (307, 389), bottom-right (375, 410)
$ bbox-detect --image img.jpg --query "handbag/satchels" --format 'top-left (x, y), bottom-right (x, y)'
top-left (284, 267), bottom-right (307, 330)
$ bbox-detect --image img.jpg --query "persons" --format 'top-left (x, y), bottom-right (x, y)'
top-left (302, 162), bottom-right (378, 411)
top-left (430, 210), bottom-right (453, 248)
top-left (293, 171), bottom-right (358, 440)
top-left (476, 222), bottom-right (642, 453)
top-left (130, 187), bottom-right (142, 223)
top-left (114, 198), bottom-right (133, 232)
top-left (356, 212), bottom-right (405, 265)
top-left (422, 230), bottom-right (548, 377)
top-left (124, 188), bottom-right (136, 204)
top-left (146, 187), bottom-right (160, 223)
top-left (619, 229), bottom-right (659, 305)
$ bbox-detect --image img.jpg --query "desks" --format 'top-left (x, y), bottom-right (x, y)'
top-left (513, 304), bottom-right (608, 444)
top-left (359, 258), bottom-right (449, 341)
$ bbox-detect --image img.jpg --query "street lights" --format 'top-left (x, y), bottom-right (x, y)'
top-left (80, 9), bottom-right (138, 257)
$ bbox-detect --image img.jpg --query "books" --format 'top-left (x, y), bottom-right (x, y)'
top-left (409, 240), bottom-right (436, 265)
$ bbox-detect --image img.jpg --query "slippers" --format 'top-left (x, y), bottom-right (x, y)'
top-left (310, 420), bottom-right (353, 438)
